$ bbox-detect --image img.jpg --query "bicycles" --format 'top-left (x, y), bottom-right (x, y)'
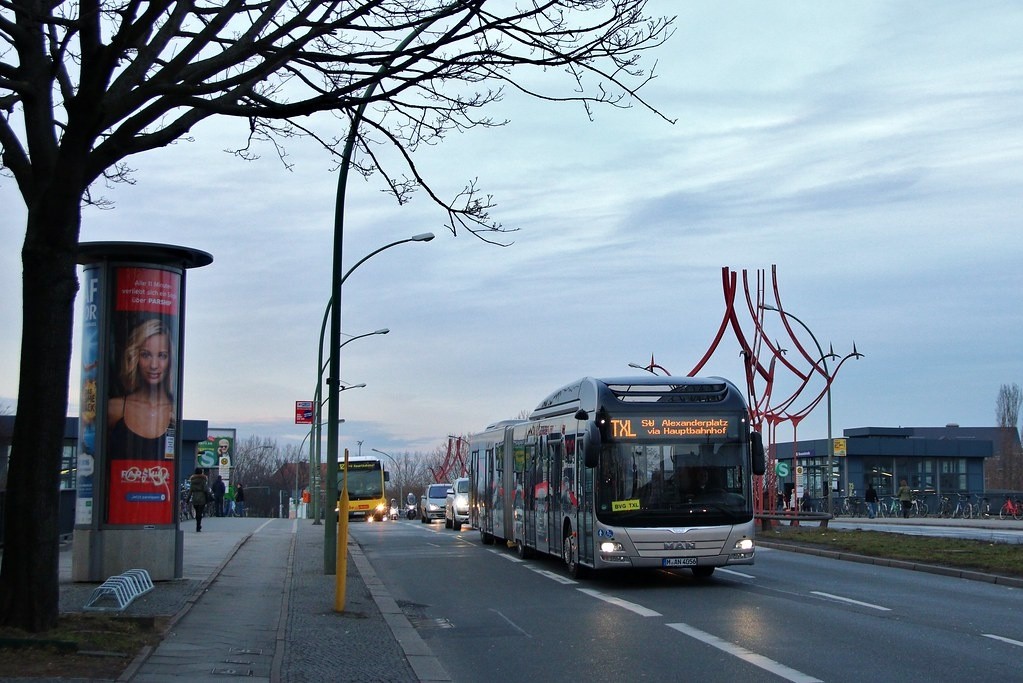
top-left (971, 493), bottom-right (990, 519)
top-left (998, 493), bottom-right (1023, 520)
top-left (950, 492), bottom-right (972, 519)
top-left (800, 494), bottom-right (951, 519)
top-left (178, 482), bottom-right (240, 523)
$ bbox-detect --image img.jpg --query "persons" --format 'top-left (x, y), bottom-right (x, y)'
top-left (802, 491), bottom-right (811, 513)
top-left (185, 468), bottom-right (209, 532)
top-left (224, 482), bottom-right (236, 517)
top-left (404, 493), bottom-right (419, 515)
top-left (898, 480), bottom-right (914, 519)
top-left (776, 490), bottom-right (785, 516)
top-left (790, 488), bottom-right (798, 510)
top-left (215, 439), bottom-right (232, 466)
top-left (389, 499), bottom-right (398, 508)
top-left (864, 484), bottom-right (879, 519)
top-left (234, 484), bottom-right (245, 516)
top-left (642, 474), bottom-right (662, 505)
top-left (212, 476), bottom-right (227, 517)
top-left (107, 318), bottom-right (175, 462)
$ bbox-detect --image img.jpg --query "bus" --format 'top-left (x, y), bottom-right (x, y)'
top-left (337, 456), bottom-right (390, 521)
top-left (465, 373), bottom-right (766, 580)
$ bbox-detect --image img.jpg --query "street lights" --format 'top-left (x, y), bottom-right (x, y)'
top-left (309, 327), bottom-right (390, 519)
top-left (757, 301), bottom-right (835, 519)
top-left (312, 231), bottom-right (435, 525)
top-left (371, 448), bottom-right (403, 511)
top-left (310, 382), bottom-right (367, 521)
top-left (294, 418), bottom-right (346, 519)
top-left (236, 445), bottom-right (273, 484)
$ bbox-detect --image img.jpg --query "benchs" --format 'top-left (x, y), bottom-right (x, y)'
top-left (81, 568), bottom-right (155, 612)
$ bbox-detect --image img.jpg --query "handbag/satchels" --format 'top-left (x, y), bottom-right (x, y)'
top-left (207, 491), bottom-right (215, 503)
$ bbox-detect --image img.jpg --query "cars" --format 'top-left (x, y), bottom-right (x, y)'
top-left (419, 483), bottom-right (454, 524)
top-left (444, 478), bottom-right (471, 532)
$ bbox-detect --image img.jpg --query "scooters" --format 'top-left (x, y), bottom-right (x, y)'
top-left (404, 503), bottom-right (417, 520)
top-left (389, 507), bottom-right (400, 520)
